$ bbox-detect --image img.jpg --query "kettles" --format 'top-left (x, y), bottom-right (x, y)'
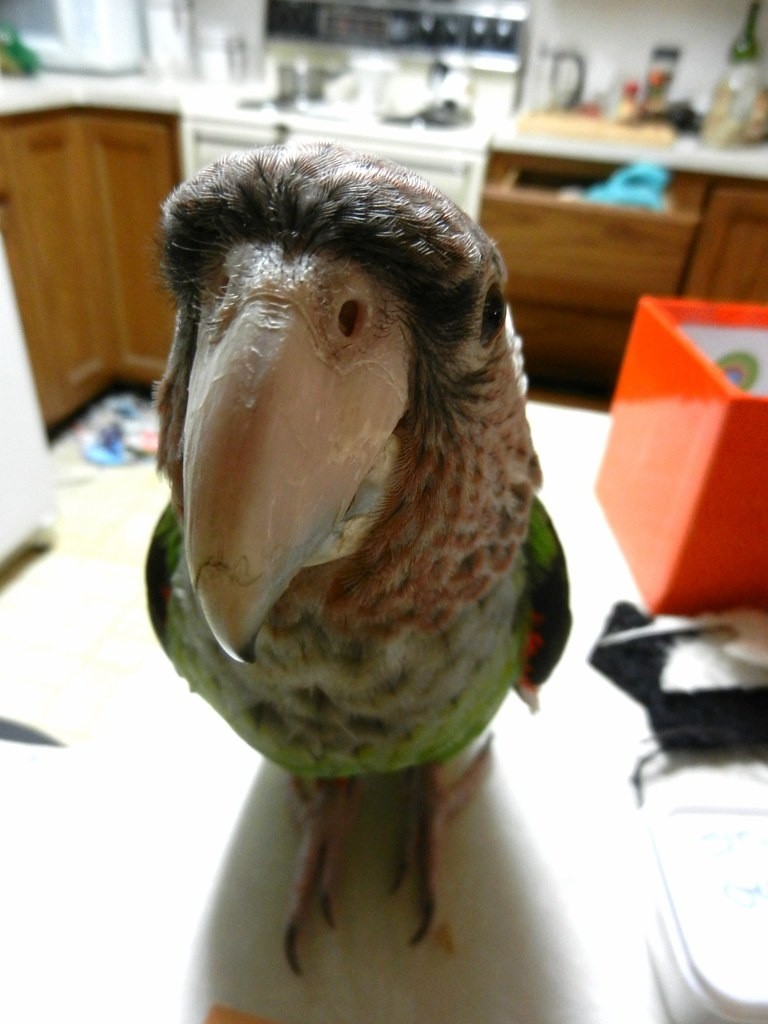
top-left (538, 49), bottom-right (588, 111)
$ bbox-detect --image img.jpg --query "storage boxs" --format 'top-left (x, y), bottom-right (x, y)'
top-left (597, 294), bottom-right (768, 615)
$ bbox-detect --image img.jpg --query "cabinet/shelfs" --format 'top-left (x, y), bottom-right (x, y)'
top-left (478, 148), bottom-right (711, 395)
top-left (676, 185), bottom-right (768, 305)
top-left (0, 108), bottom-right (179, 425)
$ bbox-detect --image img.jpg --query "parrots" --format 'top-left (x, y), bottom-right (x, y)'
top-left (139, 143), bottom-right (572, 978)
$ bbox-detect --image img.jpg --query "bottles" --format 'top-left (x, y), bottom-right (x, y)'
top-left (700, 3), bottom-right (763, 149)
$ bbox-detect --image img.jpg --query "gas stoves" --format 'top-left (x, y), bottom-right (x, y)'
top-left (204, 0), bottom-right (531, 154)
